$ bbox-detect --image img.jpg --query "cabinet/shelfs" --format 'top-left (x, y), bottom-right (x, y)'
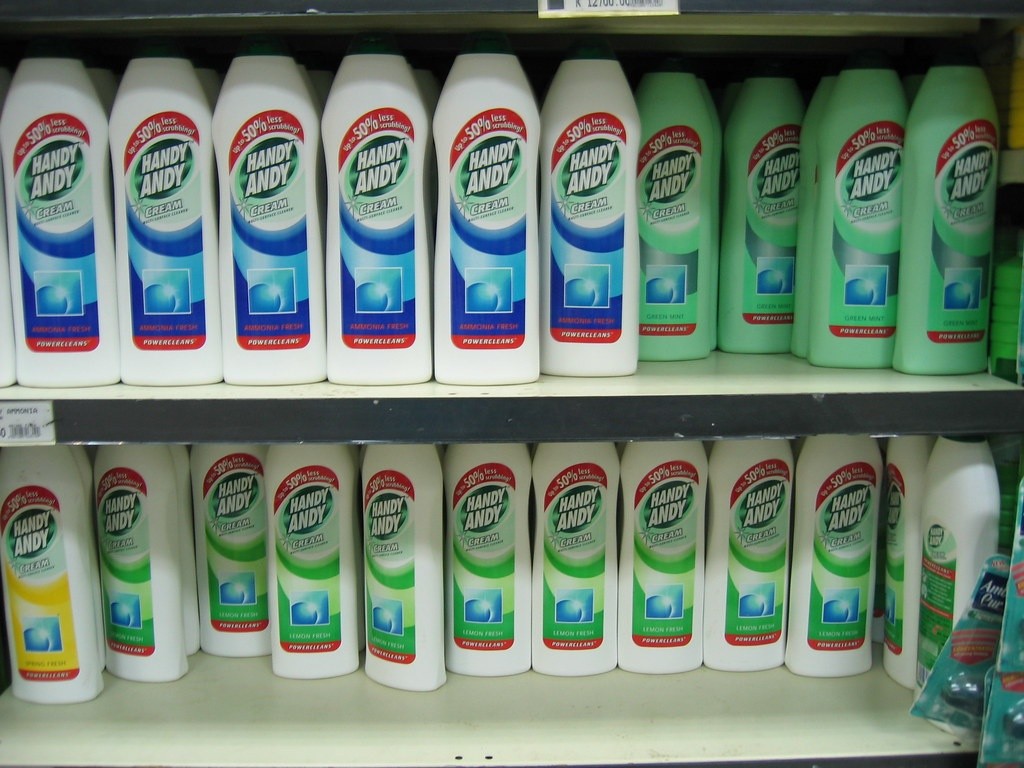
top-left (0, 0), bottom-right (1024, 768)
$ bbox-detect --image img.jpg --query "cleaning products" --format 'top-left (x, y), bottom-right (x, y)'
top-left (0, 48), bottom-right (1023, 389)
top-left (3, 432), bottom-right (1023, 763)
